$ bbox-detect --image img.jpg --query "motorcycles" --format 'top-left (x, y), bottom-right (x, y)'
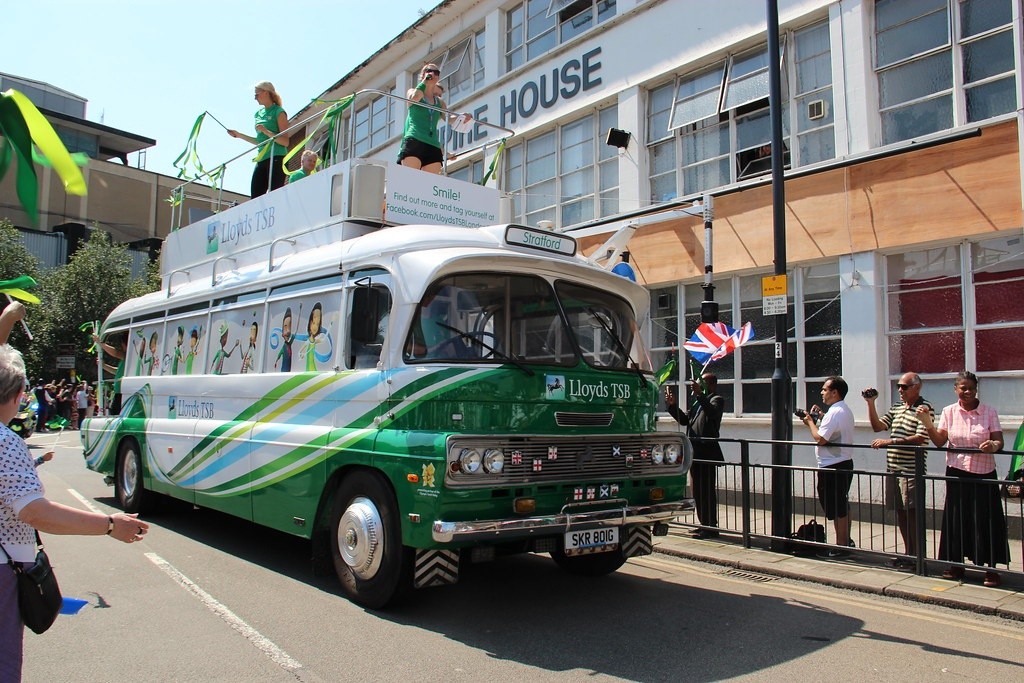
top-left (8, 393), bottom-right (38, 440)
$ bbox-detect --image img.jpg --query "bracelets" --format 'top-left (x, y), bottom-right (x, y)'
top-left (105, 516), bottom-right (114, 535)
top-left (893, 438), bottom-right (897, 444)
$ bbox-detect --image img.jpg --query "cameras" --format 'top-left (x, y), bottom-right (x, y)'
top-left (795, 408), bottom-right (819, 426)
top-left (862, 389), bottom-right (877, 398)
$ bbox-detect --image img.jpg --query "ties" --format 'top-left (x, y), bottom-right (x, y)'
top-left (697, 405), bottom-right (701, 413)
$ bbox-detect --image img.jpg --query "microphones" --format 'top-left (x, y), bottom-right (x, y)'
top-left (426, 75), bottom-right (432, 80)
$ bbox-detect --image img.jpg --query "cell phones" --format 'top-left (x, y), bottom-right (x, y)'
top-left (666, 386), bottom-right (670, 397)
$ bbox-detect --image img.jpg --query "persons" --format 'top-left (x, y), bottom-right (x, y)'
top-left (376, 282), bottom-right (452, 362)
top-left (664, 373), bottom-right (725, 539)
top-left (0, 301), bottom-right (148, 683)
top-left (800, 377), bottom-right (854, 558)
top-left (915, 371), bottom-right (1011, 586)
top-left (227, 81), bottom-right (317, 199)
top-left (865, 372), bottom-right (934, 571)
top-left (397, 64), bottom-right (472, 175)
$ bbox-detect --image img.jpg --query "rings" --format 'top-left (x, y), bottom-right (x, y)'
top-left (132, 535), bottom-right (137, 540)
top-left (137, 527), bottom-right (142, 535)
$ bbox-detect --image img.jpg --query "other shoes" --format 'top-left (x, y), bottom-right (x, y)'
top-left (885, 557), bottom-right (926, 571)
top-left (942, 566), bottom-right (964, 578)
top-left (689, 527), bottom-right (720, 539)
top-left (984, 572), bottom-right (1000, 586)
top-left (818, 539), bottom-right (855, 557)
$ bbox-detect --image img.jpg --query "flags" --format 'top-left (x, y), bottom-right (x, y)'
top-left (681, 321), bottom-right (755, 366)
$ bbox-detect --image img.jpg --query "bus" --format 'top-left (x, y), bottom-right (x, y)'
top-left (79, 88), bottom-right (697, 611)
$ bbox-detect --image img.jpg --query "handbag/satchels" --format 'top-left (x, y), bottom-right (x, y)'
top-left (797, 520), bottom-right (826, 556)
top-left (17, 550), bottom-right (63, 635)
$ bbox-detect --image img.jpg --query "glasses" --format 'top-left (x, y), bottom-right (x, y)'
top-left (254, 90), bottom-right (264, 98)
top-left (896, 383), bottom-right (915, 390)
top-left (427, 68), bottom-right (441, 76)
